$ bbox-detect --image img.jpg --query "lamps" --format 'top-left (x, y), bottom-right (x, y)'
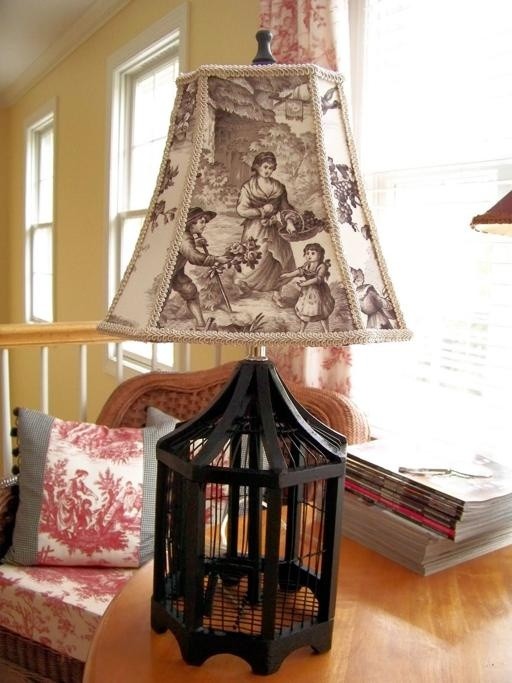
top-left (94, 29), bottom-right (416, 678)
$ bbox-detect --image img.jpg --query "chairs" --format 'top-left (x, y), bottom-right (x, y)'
top-left (0, 359), bottom-right (369, 682)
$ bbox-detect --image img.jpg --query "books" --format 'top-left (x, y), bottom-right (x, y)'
top-left (313, 437), bottom-right (511, 577)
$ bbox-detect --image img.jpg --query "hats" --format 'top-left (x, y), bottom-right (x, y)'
top-left (185, 207), bottom-right (217, 223)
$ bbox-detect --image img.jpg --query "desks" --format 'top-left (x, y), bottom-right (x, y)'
top-left (81, 498), bottom-right (512, 682)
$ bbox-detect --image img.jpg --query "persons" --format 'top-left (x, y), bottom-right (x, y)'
top-left (282, 240), bottom-right (337, 333)
top-left (163, 206), bottom-right (230, 332)
top-left (235, 148), bottom-right (297, 294)
